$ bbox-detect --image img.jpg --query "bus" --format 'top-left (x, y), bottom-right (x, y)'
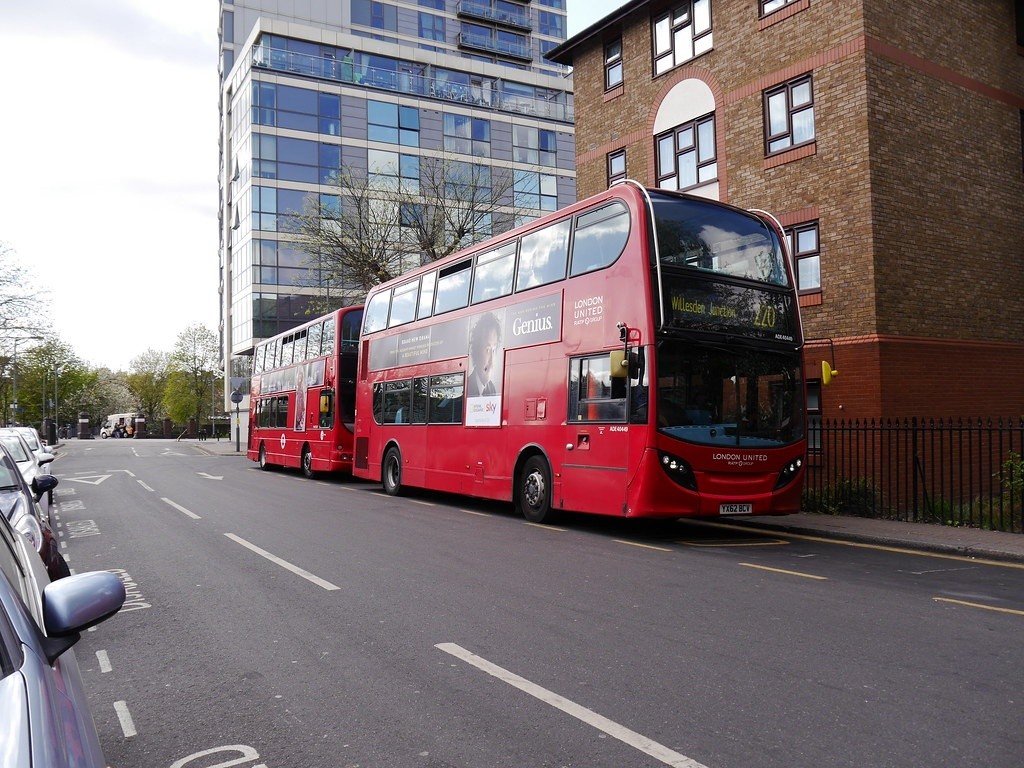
top-left (354, 178), bottom-right (840, 523)
top-left (246, 304), bottom-right (363, 478)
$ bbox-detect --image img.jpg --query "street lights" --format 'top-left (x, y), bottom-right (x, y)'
top-left (212, 373), bottom-right (225, 436)
top-left (13, 337), bottom-right (44, 427)
top-left (55, 361), bottom-right (85, 440)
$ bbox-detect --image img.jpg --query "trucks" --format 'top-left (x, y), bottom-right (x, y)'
top-left (100, 413), bottom-right (144, 439)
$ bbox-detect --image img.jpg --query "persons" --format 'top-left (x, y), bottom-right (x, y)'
top-left (466, 313), bottom-right (500, 396)
top-left (114, 423), bottom-right (120, 439)
top-left (124, 423), bottom-right (133, 438)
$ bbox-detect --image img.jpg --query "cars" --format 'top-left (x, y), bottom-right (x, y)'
top-left (0, 427), bottom-right (125, 768)
